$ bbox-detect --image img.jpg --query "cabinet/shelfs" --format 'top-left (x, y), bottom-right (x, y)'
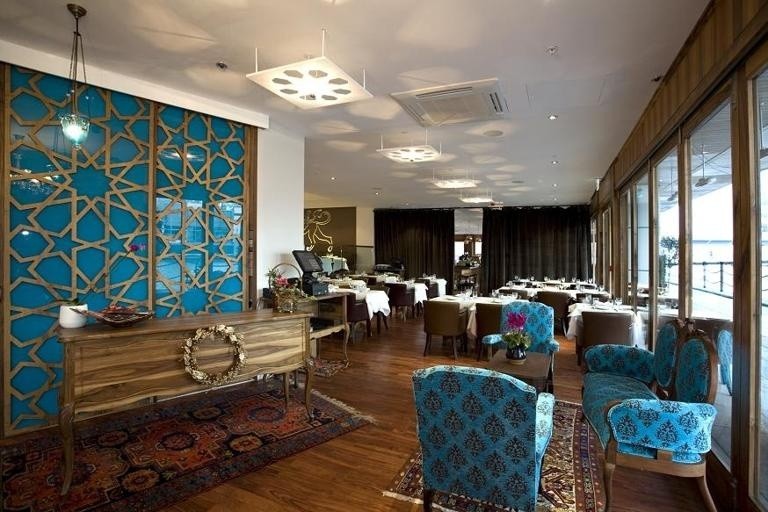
top-left (264, 290), bottom-right (351, 371)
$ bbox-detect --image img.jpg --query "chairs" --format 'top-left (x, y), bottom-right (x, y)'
top-left (411, 366), bottom-right (555, 512)
top-left (483, 301), bottom-right (558, 395)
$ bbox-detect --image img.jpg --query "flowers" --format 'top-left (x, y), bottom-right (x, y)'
top-left (501, 310), bottom-right (531, 351)
top-left (55, 244), bottom-right (145, 305)
top-left (178, 324), bottom-right (248, 388)
top-left (266, 269), bottom-right (314, 301)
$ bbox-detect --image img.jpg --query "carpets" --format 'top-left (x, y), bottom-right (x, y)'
top-left (0, 377), bottom-right (373, 512)
top-left (379, 391), bottom-right (603, 512)
top-left (298, 358), bottom-right (351, 377)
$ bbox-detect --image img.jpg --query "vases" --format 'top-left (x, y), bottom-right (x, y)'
top-left (270, 292), bottom-right (299, 314)
top-left (59, 303), bottom-right (88, 329)
top-left (505, 345), bottom-right (527, 366)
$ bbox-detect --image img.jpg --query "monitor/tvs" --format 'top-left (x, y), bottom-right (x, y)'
top-left (292, 250), bottom-right (324, 273)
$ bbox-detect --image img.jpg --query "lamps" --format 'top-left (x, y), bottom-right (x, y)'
top-left (692, 145), bottom-right (712, 187)
top-left (463, 219), bottom-right (481, 246)
top-left (756, 95), bottom-right (767, 160)
top-left (666, 151), bottom-right (678, 204)
top-left (59, 4), bottom-right (90, 153)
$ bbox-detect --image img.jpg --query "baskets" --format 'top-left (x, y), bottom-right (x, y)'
top-left (269, 263), bottom-right (302, 313)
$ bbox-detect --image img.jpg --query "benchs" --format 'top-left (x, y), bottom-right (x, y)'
top-left (580, 319), bottom-right (719, 512)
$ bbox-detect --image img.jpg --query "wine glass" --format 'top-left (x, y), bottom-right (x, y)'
top-left (491, 275), bottom-right (622, 313)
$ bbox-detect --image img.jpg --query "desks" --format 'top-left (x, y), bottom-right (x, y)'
top-left (57, 307), bottom-right (314, 495)
top-left (316, 255), bottom-right (348, 278)
